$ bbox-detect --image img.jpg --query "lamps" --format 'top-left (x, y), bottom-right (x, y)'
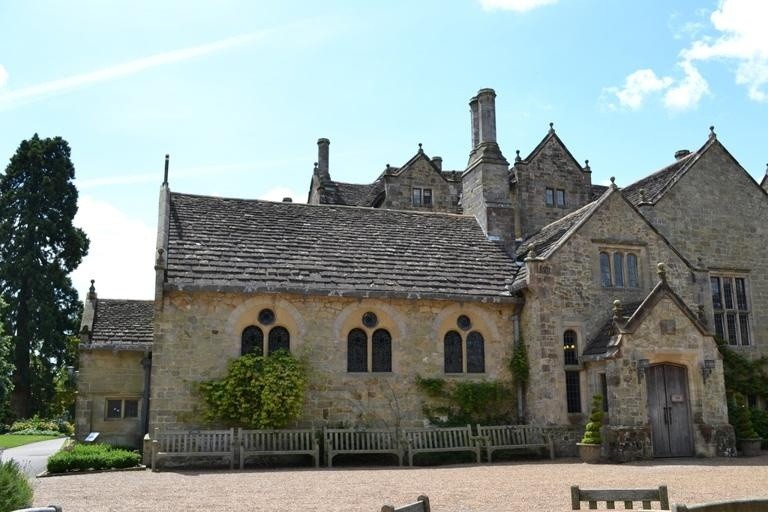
top-left (703, 360), bottom-right (715, 385)
top-left (638, 359), bottom-right (650, 383)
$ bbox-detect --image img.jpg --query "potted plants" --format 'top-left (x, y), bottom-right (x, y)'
top-left (736, 392), bottom-right (763, 457)
top-left (576, 394), bottom-right (605, 463)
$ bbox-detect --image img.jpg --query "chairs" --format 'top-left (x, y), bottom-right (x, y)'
top-left (671, 499), bottom-right (768, 512)
top-left (571, 485), bottom-right (669, 510)
top-left (381, 495), bottom-right (430, 512)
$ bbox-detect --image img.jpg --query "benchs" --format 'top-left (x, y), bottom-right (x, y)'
top-left (153, 427), bottom-right (236, 470)
top-left (403, 424), bottom-right (480, 467)
top-left (237, 427), bottom-right (320, 468)
top-left (322, 426), bottom-right (405, 469)
top-left (477, 423), bottom-right (555, 463)
